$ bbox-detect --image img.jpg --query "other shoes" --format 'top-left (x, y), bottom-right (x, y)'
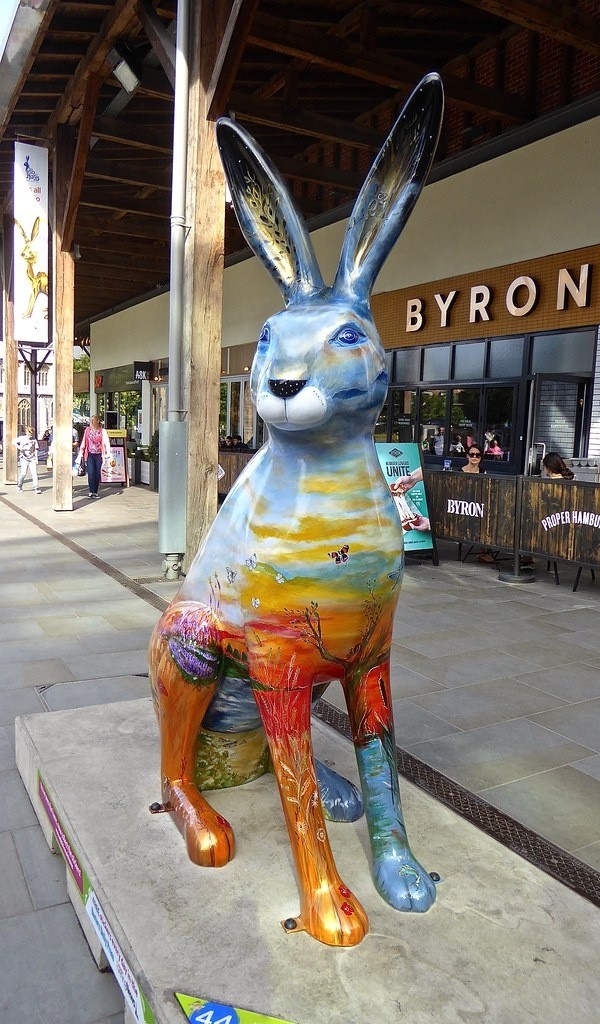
top-left (477, 553), bottom-right (495, 563)
top-left (87, 492), bottom-right (93, 498)
top-left (18, 486), bottom-right (24, 492)
top-left (93, 493), bottom-right (101, 499)
top-left (35, 488), bottom-right (41, 493)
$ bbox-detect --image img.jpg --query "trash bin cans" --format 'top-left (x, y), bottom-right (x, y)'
top-left (126, 453), bottom-right (136, 486)
top-left (139, 458), bottom-right (155, 490)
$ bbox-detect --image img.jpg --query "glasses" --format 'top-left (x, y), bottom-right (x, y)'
top-left (468, 453), bottom-right (482, 458)
top-left (541, 464), bottom-right (545, 469)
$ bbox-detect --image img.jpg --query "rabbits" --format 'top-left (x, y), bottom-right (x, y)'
top-left (148, 73), bottom-right (445, 945)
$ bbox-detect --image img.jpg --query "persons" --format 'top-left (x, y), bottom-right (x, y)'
top-left (12, 426), bottom-right (41, 494)
top-left (419, 427), bottom-right (503, 460)
top-left (391, 466), bottom-right (431, 532)
top-left (520, 453), bottom-right (577, 569)
top-left (18, 422), bottom-right (83, 498)
top-left (219, 435), bottom-right (253, 449)
top-left (78, 414), bottom-right (115, 499)
top-left (126, 429), bottom-right (137, 453)
top-left (457, 444), bottom-right (495, 562)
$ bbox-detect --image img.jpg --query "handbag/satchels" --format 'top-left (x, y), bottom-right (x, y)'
top-left (77, 457), bottom-right (86, 476)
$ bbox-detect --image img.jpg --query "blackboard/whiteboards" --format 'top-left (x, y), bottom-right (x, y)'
top-left (37, 440), bottom-right (48, 462)
